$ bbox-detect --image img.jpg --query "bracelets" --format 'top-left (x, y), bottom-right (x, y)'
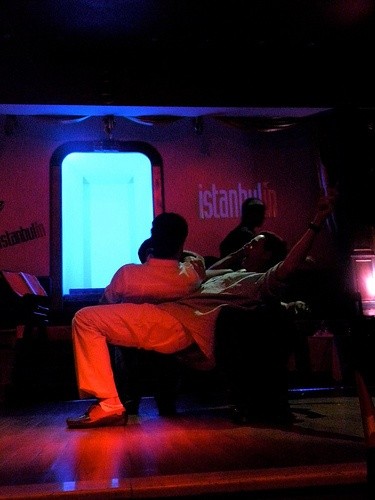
top-left (307, 222), bottom-right (322, 234)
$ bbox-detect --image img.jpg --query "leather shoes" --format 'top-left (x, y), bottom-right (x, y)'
top-left (66, 403), bottom-right (129, 428)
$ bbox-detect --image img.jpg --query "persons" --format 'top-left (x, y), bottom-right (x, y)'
top-left (66, 188), bottom-right (337, 428)
top-left (219, 198), bottom-right (266, 271)
top-left (99, 211), bottom-right (205, 412)
